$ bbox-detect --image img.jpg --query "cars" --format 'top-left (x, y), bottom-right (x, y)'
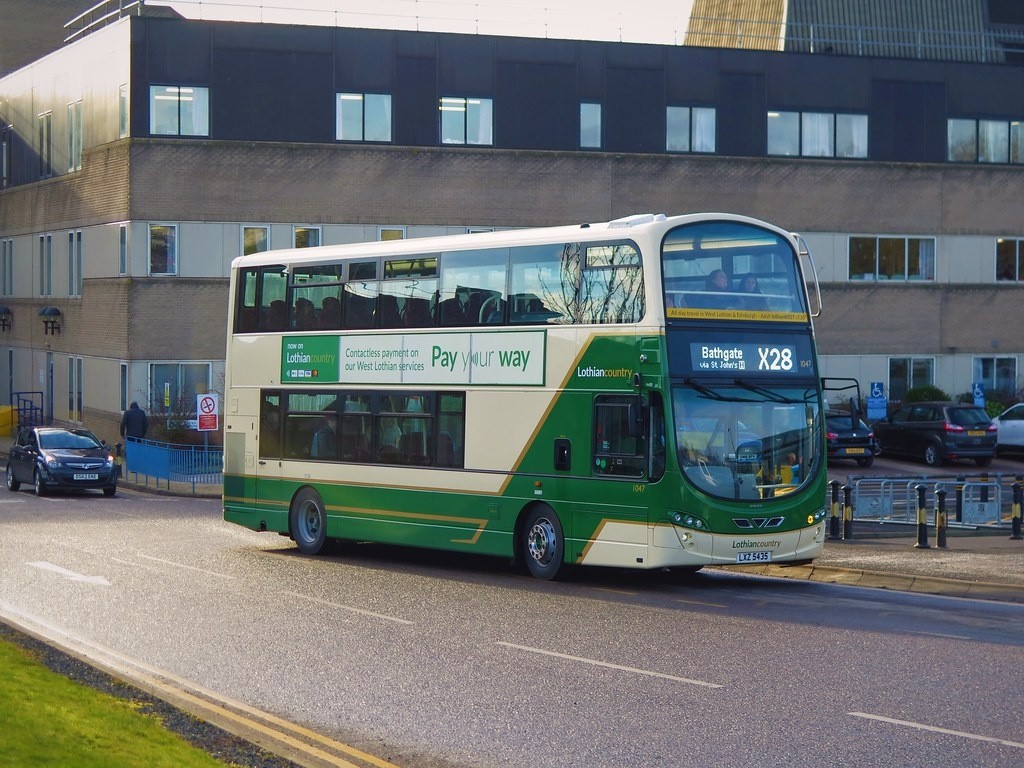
top-left (677, 417), bottom-right (762, 466)
top-left (825, 408), bottom-right (877, 468)
top-left (991, 403), bottom-right (1024, 455)
top-left (7, 424), bottom-right (118, 497)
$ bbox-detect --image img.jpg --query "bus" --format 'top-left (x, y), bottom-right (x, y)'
top-left (221, 211), bottom-right (830, 583)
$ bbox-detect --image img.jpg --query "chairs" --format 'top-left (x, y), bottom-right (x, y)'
top-left (265, 292), bottom-right (707, 333)
top-left (288, 418), bottom-right (457, 467)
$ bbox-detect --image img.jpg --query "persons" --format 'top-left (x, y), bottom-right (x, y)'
top-left (120, 401), bottom-right (148, 444)
top-left (699, 269), bottom-right (771, 312)
top-left (312, 416), bottom-right (338, 460)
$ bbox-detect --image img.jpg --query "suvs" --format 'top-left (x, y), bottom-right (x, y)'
top-left (869, 401), bottom-right (999, 468)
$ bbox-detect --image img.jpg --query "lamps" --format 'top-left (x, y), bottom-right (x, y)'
top-left (0, 307), bottom-right (12, 331)
top-left (39, 306), bottom-right (61, 336)
